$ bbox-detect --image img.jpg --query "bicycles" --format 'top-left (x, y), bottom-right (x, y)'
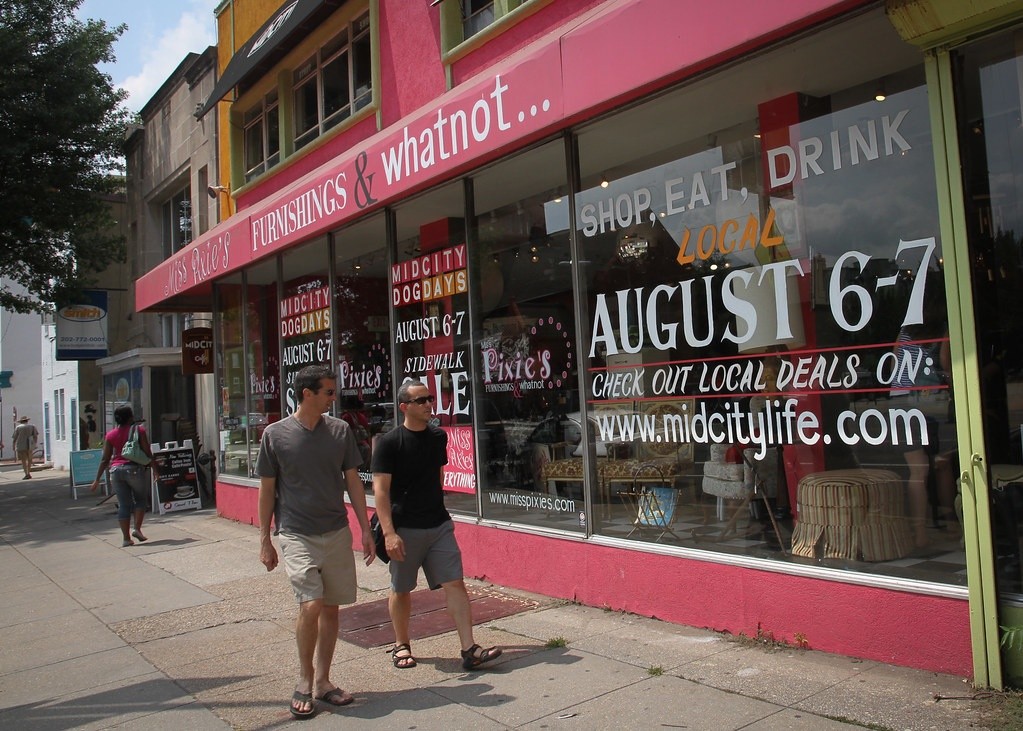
top-left (32, 443), bottom-right (44, 466)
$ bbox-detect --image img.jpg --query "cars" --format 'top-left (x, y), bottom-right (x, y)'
top-left (359, 403), bottom-right (437, 434)
top-left (484, 408), bottom-right (689, 498)
top-left (228, 412), bottom-right (268, 442)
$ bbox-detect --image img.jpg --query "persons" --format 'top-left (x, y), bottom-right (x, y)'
top-left (254, 365), bottom-right (376, 716)
top-left (11, 416), bottom-right (39, 480)
top-left (91, 405), bottom-right (161, 547)
top-left (370, 378), bottom-right (503, 671)
top-left (892, 277), bottom-right (950, 554)
top-left (759, 356), bottom-right (795, 442)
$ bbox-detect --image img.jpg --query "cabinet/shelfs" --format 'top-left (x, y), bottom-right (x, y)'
top-left (601, 346), bottom-right (671, 398)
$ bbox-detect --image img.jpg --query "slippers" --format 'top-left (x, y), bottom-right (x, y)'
top-left (316, 687), bottom-right (353, 705)
top-left (289, 684), bottom-right (315, 715)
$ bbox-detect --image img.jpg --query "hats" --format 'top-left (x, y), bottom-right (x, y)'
top-left (17, 416), bottom-right (29, 422)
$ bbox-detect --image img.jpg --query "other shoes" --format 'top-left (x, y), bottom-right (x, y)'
top-left (132, 529), bottom-right (147, 541)
top-left (22, 475), bottom-right (32, 480)
top-left (122, 539), bottom-right (133, 547)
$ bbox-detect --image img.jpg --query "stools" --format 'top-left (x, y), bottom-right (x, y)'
top-left (791, 468), bottom-right (917, 563)
top-left (950, 463), bottom-right (1023, 579)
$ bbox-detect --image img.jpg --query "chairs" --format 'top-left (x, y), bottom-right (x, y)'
top-left (541, 398), bottom-right (696, 526)
top-left (701, 417), bottom-right (805, 556)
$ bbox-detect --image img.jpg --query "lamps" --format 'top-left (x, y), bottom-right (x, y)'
top-left (598, 174), bottom-right (608, 188)
top-left (207, 181), bottom-right (231, 199)
top-left (704, 132), bottom-right (719, 153)
top-left (733, 256), bottom-right (810, 440)
top-left (493, 235), bottom-right (554, 264)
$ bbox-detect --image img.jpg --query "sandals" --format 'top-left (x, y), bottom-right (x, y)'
top-left (460, 644), bottom-right (503, 669)
top-left (387, 643), bottom-right (416, 668)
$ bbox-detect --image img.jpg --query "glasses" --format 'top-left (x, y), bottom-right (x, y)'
top-left (404, 395), bottom-right (435, 405)
top-left (309, 387), bottom-right (336, 396)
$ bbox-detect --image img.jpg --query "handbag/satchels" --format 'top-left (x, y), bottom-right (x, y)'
top-left (121, 424), bottom-right (151, 466)
top-left (28, 426), bottom-right (36, 450)
top-left (370, 511), bottom-right (392, 565)
